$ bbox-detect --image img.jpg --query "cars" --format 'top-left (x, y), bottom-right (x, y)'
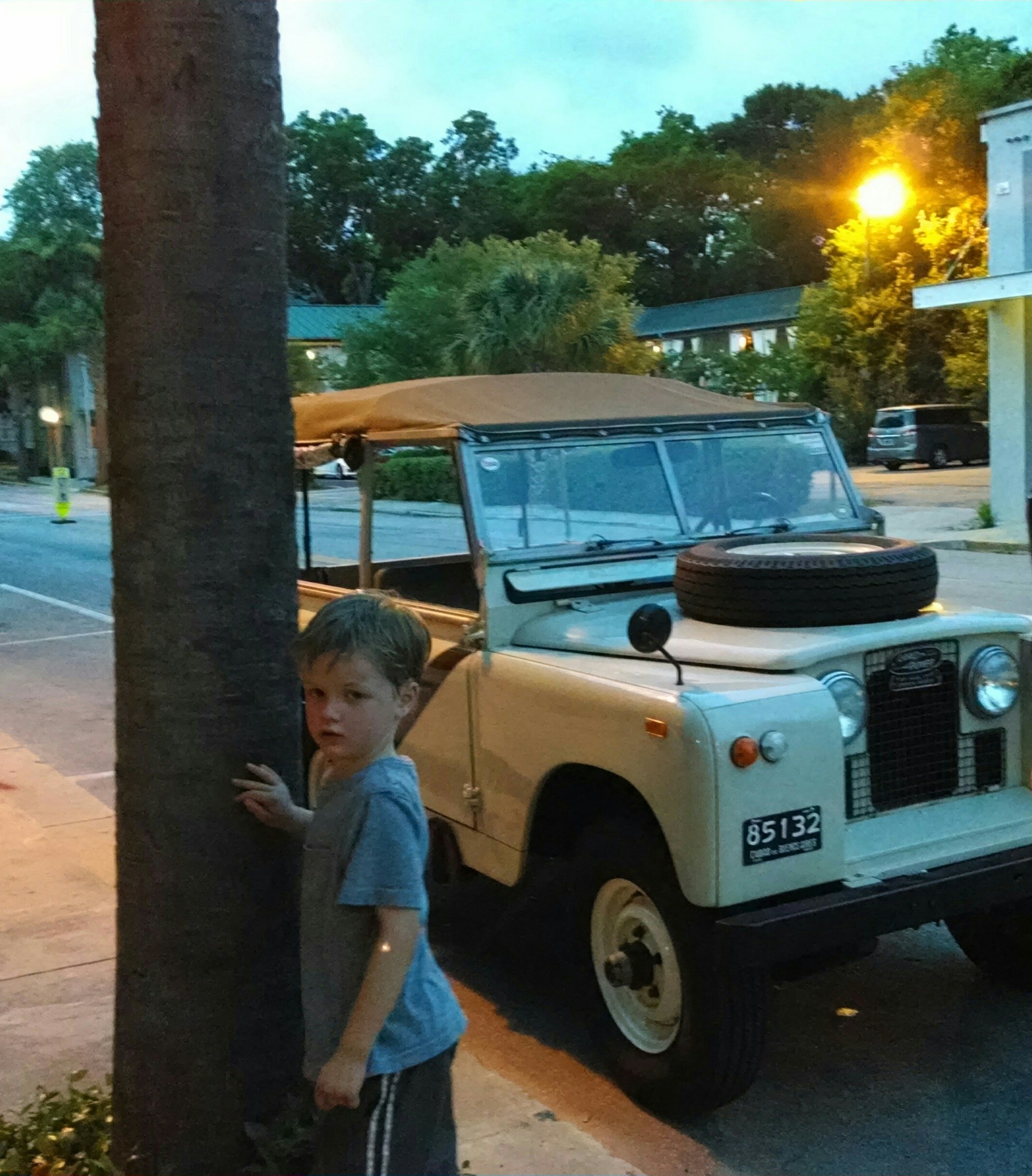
top-left (312, 458), bottom-right (358, 480)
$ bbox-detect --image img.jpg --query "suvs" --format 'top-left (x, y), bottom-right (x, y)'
top-left (292, 371), bottom-right (1032, 1120)
top-left (866, 405), bottom-right (989, 468)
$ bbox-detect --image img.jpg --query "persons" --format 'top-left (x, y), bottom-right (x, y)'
top-left (231, 595), bottom-right (468, 1176)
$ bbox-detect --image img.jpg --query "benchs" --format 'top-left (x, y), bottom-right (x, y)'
top-left (373, 563), bottom-right (480, 613)
